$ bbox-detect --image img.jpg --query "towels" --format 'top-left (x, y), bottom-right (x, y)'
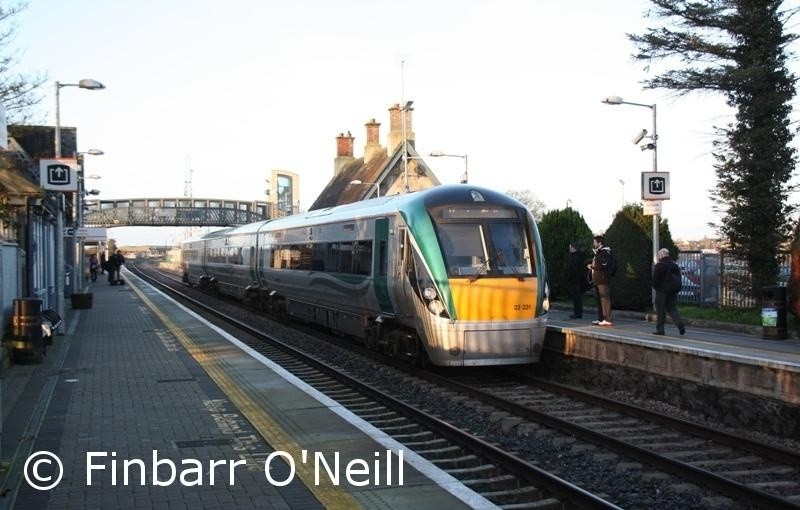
top-left (762, 286), bottom-right (787, 340)
top-left (64, 263), bottom-right (74, 299)
top-left (12, 298), bottom-right (43, 365)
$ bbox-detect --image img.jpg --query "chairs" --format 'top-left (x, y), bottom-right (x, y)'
top-left (591, 317), bottom-right (613, 327)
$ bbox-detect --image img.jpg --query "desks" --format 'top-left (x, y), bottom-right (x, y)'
top-left (431, 152), bottom-right (468, 185)
top-left (350, 180), bottom-right (379, 197)
top-left (55, 80), bottom-right (104, 335)
top-left (601, 95), bottom-right (670, 311)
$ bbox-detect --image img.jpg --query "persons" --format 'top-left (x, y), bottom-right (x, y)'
top-left (101, 251), bottom-right (106, 275)
top-left (90, 253), bottom-right (97, 282)
top-left (114, 249), bottom-right (125, 284)
top-left (652, 247), bottom-right (686, 335)
top-left (567, 242), bottom-right (585, 318)
top-left (586, 235), bottom-right (614, 327)
top-left (108, 253), bottom-right (116, 284)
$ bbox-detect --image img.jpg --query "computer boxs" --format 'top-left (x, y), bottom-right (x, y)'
top-left (633, 129), bottom-right (648, 144)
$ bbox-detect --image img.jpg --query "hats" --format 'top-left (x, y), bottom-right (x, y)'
top-left (725, 263), bottom-right (791, 299)
top-left (678, 259), bottom-right (701, 301)
